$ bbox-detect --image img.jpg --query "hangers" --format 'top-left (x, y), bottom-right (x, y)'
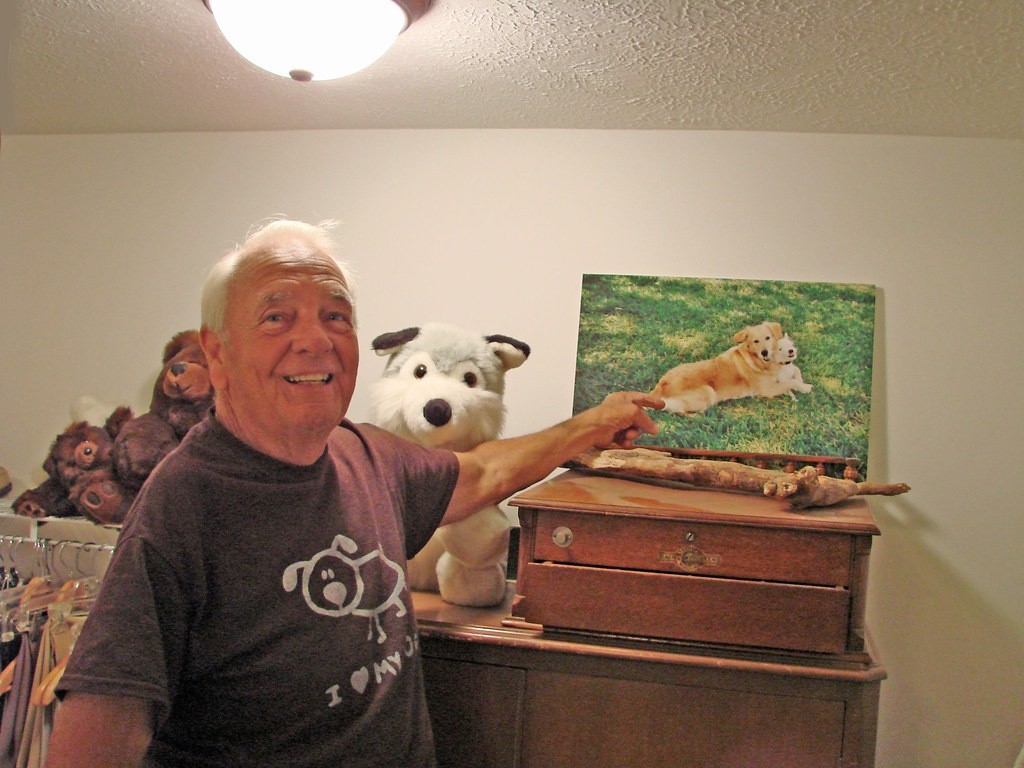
top-left (0, 534), bottom-right (108, 642)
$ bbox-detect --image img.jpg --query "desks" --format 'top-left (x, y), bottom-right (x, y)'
top-left (411, 591), bottom-right (887, 768)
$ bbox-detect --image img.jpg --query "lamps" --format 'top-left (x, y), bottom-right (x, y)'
top-left (202, 0), bottom-right (434, 83)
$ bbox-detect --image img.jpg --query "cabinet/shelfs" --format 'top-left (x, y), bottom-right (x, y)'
top-left (506, 469), bottom-right (881, 665)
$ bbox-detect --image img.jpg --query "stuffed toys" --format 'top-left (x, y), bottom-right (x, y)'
top-left (366, 325), bottom-right (531, 607)
top-left (70, 330), bottom-right (214, 525)
top-left (12, 406), bottom-right (132, 518)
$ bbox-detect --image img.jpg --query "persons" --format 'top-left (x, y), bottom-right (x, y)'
top-left (44, 220), bottom-right (665, 768)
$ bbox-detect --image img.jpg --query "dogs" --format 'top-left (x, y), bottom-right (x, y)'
top-left (649, 321), bottom-right (813, 415)
